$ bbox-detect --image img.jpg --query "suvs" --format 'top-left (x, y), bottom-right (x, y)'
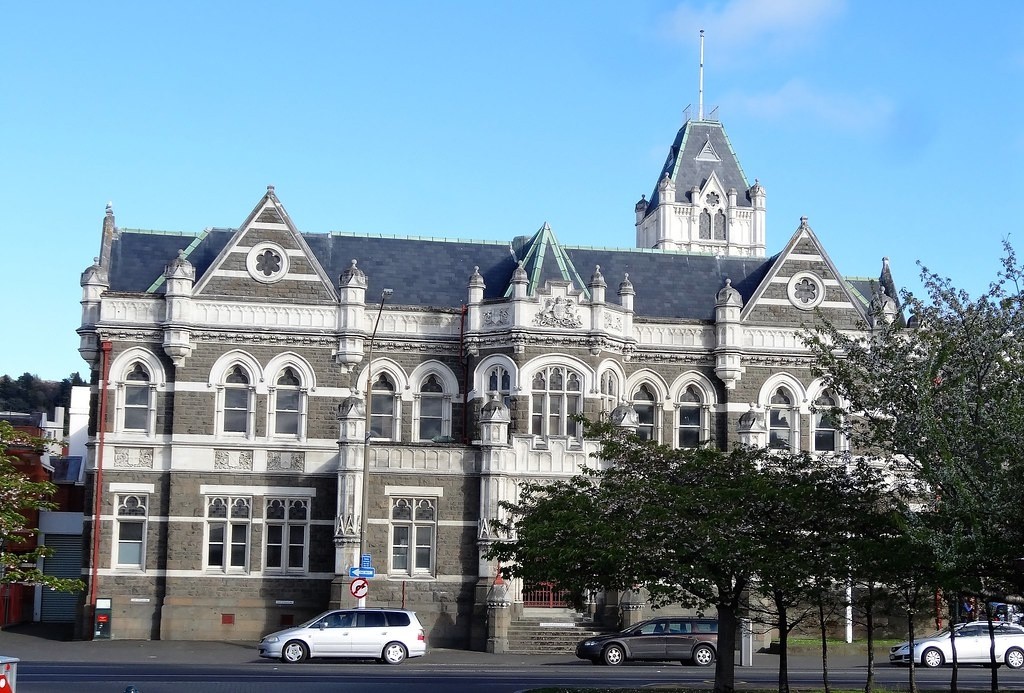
top-left (889, 621), bottom-right (1024, 670)
top-left (257, 606), bottom-right (426, 665)
top-left (575, 616), bottom-right (718, 667)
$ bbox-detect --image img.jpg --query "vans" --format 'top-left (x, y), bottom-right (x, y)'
top-left (977, 603), bottom-right (1017, 622)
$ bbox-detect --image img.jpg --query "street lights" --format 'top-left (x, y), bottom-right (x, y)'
top-left (358, 289), bottom-right (395, 610)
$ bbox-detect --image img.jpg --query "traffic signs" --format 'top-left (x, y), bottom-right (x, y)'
top-left (349, 567), bottom-right (375, 578)
